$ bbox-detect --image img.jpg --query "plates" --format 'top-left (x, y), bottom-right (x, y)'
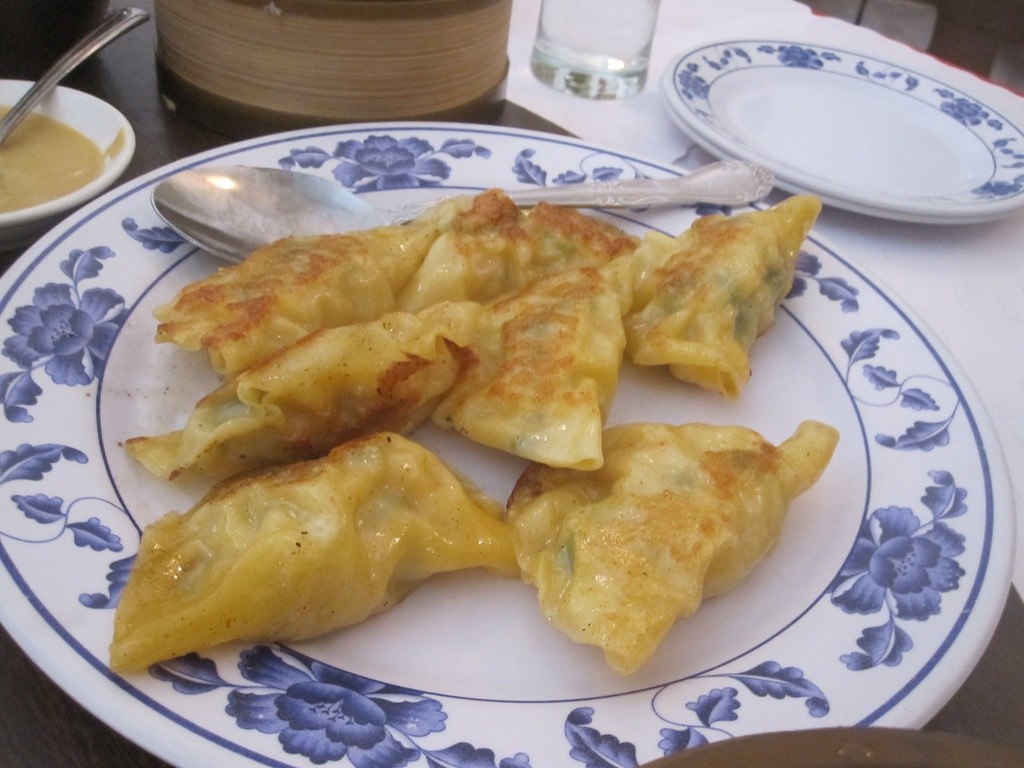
top-left (0, 121), bottom-right (1017, 768)
top-left (655, 33), bottom-right (1024, 227)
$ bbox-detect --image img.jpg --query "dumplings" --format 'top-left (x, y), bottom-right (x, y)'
top-left (110, 188), bottom-right (843, 676)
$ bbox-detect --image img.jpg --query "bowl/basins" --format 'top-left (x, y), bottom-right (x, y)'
top-left (0, 78), bottom-right (137, 256)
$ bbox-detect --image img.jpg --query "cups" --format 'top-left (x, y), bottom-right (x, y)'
top-left (530, 0), bottom-right (661, 100)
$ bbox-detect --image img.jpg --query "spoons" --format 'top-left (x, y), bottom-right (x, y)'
top-left (150, 164), bottom-right (777, 264)
